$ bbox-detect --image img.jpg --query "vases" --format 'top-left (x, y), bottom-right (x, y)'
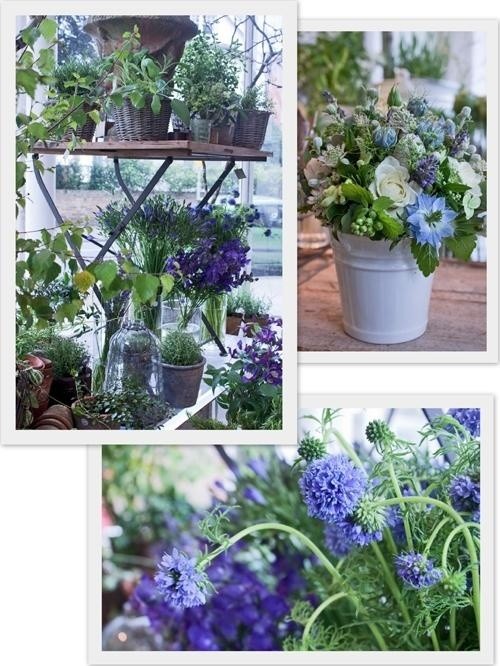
top-left (330, 226), bottom-right (437, 345)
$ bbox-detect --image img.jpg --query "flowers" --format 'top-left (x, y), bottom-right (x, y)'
top-left (298, 82), bottom-right (487, 277)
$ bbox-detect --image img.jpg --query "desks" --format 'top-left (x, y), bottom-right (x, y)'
top-left (27, 140), bottom-right (272, 356)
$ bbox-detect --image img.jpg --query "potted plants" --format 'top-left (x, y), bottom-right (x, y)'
top-left (16, 15), bottom-right (281, 149)
top-left (16, 326), bottom-right (206, 429)
